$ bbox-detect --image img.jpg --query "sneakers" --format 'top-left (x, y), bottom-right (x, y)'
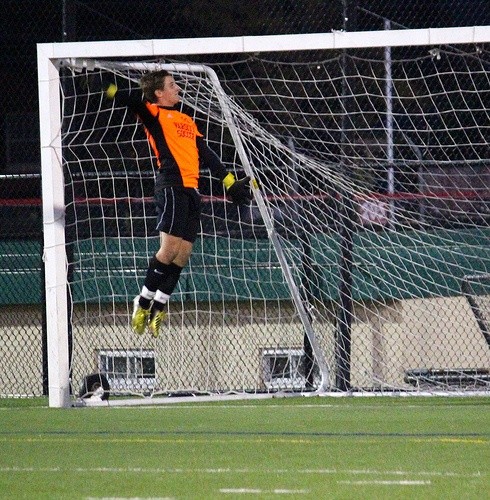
top-left (132, 296), bottom-right (152, 336)
top-left (148, 300), bottom-right (167, 338)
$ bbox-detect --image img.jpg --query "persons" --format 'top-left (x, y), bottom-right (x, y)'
top-left (82, 68), bottom-right (254, 339)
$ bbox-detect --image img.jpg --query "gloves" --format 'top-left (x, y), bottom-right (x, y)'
top-left (222, 171), bottom-right (253, 210)
top-left (94, 68), bottom-right (118, 98)
